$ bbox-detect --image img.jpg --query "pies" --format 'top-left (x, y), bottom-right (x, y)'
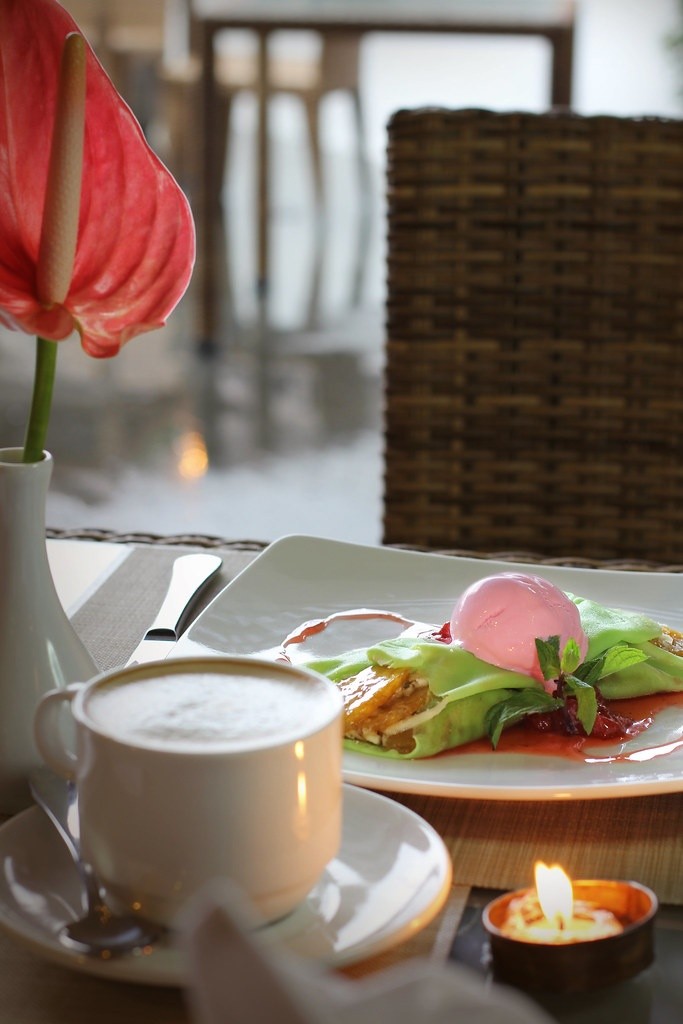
top-left (304, 588), bottom-right (683, 757)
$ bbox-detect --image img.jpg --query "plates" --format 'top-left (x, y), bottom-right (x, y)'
top-left (0, 783), bottom-right (453, 987)
top-left (163, 536), bottom-right (683, 800)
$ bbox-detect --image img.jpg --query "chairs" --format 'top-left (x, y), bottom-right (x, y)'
top-left (150, 0), bottom-right (374, 335)
top-left (379, 100), bottom-right (683, 566)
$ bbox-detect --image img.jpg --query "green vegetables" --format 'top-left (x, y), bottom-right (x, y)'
top-left (485, 637), bottom-right (605, 750)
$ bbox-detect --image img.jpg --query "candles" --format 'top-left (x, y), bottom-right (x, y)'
top-left (482, 858), bottom-right (657, 995)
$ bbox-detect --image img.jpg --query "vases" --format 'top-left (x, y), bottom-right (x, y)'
top-left (1, 449), bottom-right (100, 818)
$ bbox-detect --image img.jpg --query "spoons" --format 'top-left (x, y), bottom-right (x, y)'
top-left (29, 770), bottom-right (165, 957)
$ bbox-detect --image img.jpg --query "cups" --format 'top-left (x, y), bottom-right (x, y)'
top-left (34, 656), bottom-right (344, 932)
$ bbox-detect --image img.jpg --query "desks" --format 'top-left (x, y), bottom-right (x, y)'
top-left (188, 1), bottom-right (579, 358)
top-left (0, 526), bottom-right (683, 1024)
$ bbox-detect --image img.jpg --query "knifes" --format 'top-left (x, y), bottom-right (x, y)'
top-left (124, 553), bottom-right (224, 668)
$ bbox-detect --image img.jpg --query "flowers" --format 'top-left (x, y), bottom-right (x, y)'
top-left (0, 0), bottom-right (198, 464)
